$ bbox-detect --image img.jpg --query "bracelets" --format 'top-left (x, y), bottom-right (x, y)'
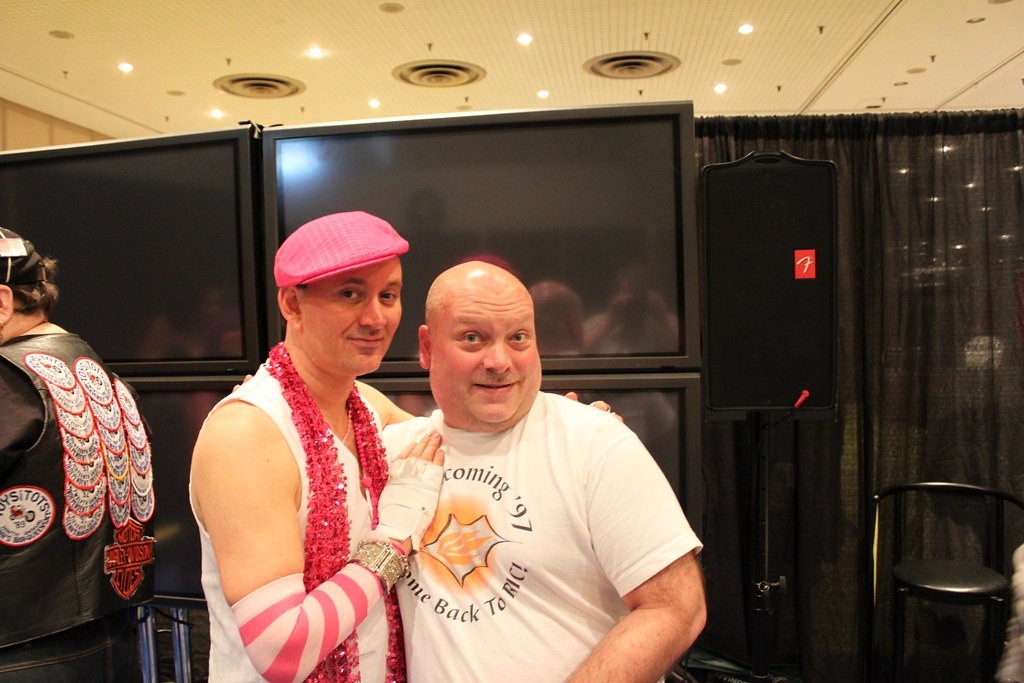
top-left (347, 540), bottom-right (409, 597)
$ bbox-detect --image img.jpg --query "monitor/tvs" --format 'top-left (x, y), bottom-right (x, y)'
top-left (0, 100), bottom-right (702, 610)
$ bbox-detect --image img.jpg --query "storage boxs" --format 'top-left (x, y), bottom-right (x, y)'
top-left (0, 100), bottom-right (703, 620)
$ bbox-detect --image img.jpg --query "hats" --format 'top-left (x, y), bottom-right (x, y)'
top-left (274, 211), bottom-right (408, 288)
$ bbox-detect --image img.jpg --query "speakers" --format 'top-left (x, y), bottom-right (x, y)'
top-left (699, 148), bottom-right (838, 411)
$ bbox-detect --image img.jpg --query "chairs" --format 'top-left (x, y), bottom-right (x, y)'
top-left (869, 482), bottom-right (1024, 683)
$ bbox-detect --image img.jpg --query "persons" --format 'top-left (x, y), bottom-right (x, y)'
top-left (0, 228), bottom-right (154, 683)
top-left (188, 210), bottom-right (625, 683)
top-left (383, 260), bottom-right (706, 683)
top-left (533, 261), bottom-right (679, 476)
